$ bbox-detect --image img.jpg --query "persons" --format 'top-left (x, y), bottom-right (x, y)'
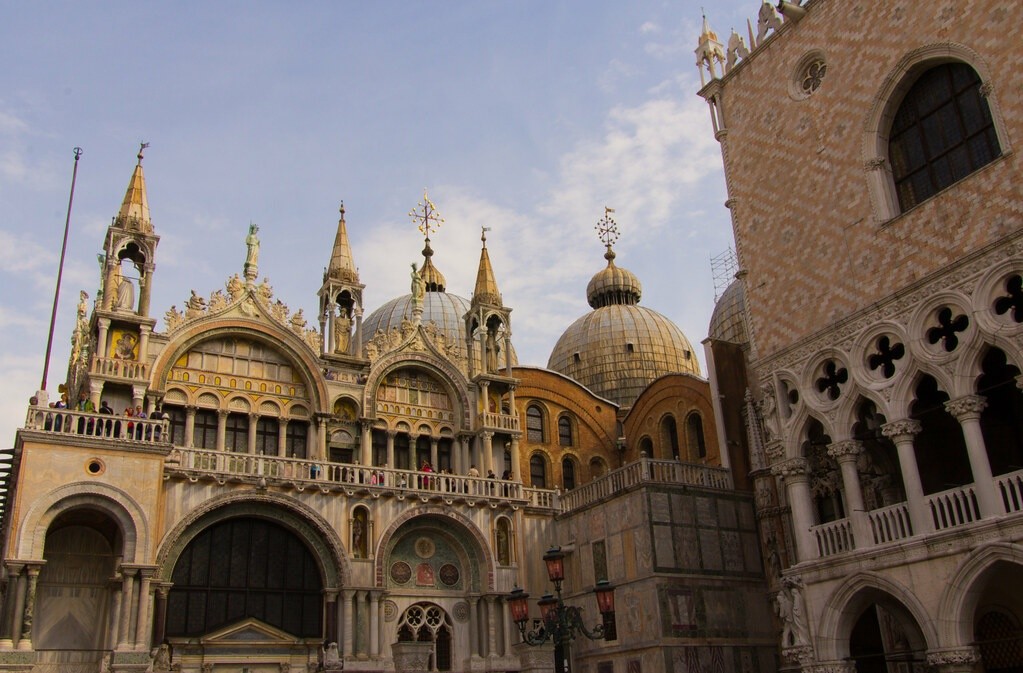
top-left (370, 460), bottom-right (513, 496)
top-left (310, 455), bottom-right (320, 479)
top-left (29, 391), bottom-right (163, 442)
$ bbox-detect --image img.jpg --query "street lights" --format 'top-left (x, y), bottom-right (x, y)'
top-left (505, 544), bottom-right (617, 673)
